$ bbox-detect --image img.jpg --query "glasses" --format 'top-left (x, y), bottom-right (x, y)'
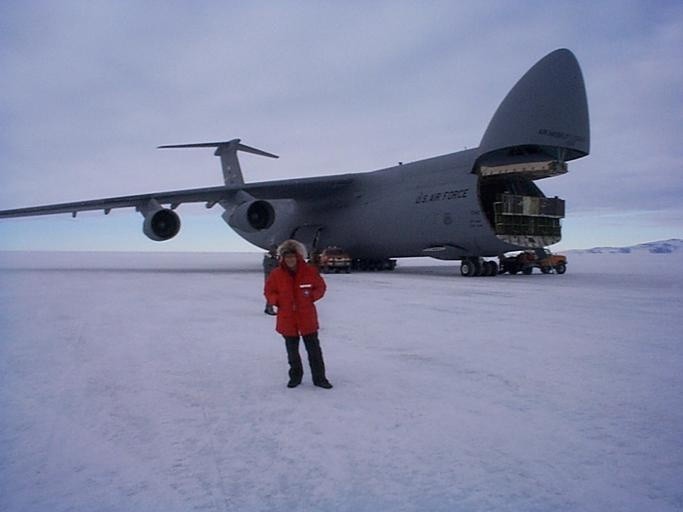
top-left (284, 254), bottom-right (295, 258)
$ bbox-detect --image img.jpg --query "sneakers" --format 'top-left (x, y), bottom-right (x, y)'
top-left (265, 308), bottom-right (276, 315)
top-left (289, 377), bottom-right (301, 386)
top-left (314, 377), bottom-right (332, 388)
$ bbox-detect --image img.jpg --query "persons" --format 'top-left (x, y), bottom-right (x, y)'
top-left (262, 238), bottom-right (333, 390)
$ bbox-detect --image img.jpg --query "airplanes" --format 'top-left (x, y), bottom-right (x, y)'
top-left (1, 47), bottom-right (593, 278)
